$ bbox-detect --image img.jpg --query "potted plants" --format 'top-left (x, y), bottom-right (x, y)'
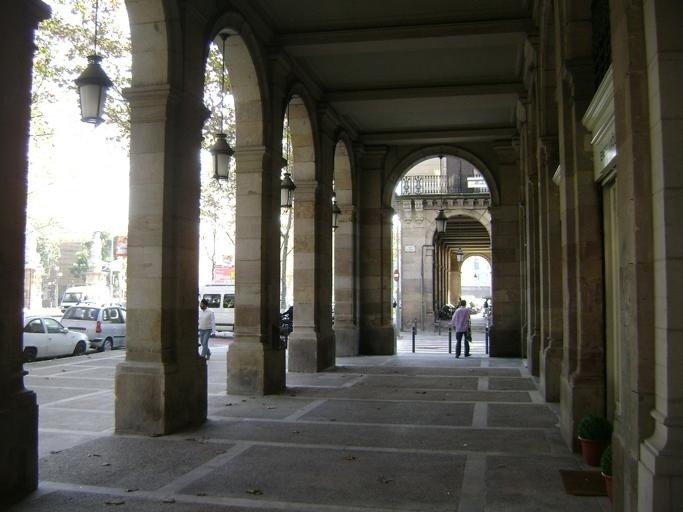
top-left (575, 411), bottom-right (614, 466)
top-left (602, 442), bottom-right (613, 504)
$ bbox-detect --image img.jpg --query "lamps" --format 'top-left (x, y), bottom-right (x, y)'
top-left (434, 154), bottom-right (448, 233)
top-left (330, 199), bottom-right (341, 228)
top-left (208, 32), bottom-right (235, 181)
top-left (457, 247), bottom-right (463, 262)
top-left (73, 0), bottom-right (112, 125)
top-left (279, 100), bottom-right (297, 208)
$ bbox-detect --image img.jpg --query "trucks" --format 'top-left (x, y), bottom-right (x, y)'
top-left (60, 285), bottom-right (112, 315)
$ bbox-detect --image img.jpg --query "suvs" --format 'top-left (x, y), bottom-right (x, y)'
top-left (58, 300), bottom-right (129, 353)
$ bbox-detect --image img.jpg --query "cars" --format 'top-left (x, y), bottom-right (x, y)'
top-left (22, 316), bottom-right (92, 363)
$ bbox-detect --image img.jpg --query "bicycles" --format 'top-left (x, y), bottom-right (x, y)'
top-left (280, 312), bottom-right (291, 348)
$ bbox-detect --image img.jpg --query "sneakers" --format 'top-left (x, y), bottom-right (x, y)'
top-left (207, 353), bottom-right (211, 359)
top-left (465, 353), bottom-right (470, 357)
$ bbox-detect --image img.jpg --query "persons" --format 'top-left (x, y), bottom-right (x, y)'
top-left (450, 299), bottom-right (472, 360)
top-left (198, 297), bottom-right (216, 361)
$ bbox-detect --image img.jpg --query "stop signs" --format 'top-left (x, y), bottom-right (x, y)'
top-left (393, 269), bottom-right (399, 281)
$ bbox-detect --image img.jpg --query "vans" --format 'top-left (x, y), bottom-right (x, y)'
top-left (198, 282), bottom-right (235, 333)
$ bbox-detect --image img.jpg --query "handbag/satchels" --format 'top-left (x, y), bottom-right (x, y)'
top-left (467, 327), bottom-right (472, 342)
